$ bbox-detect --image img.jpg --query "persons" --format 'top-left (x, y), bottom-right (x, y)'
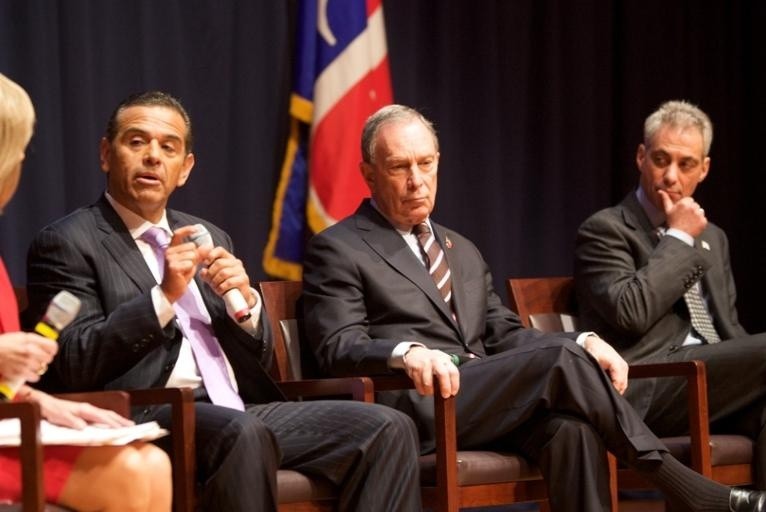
top-left (0, 71), bottom-right (175, 511)
top-left (571, 101), bottom-right (766, 493)
top-left (24, 91), bottom-right (422, 511)
top-left (295, 104), bottom-right (765, 511)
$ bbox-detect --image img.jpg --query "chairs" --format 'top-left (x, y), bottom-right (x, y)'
top-left (1, 274), bottom-right (765, 511)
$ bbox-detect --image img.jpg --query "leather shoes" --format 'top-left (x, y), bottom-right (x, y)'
top-left (728, 484), bottom-right (766, 512)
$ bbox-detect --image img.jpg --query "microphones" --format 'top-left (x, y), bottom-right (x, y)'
top-left (185, 220), bottom-right (252, 329)
top-left (0, 289), bottom-right (82, 408)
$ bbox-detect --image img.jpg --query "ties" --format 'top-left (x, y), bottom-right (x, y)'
top-left (141, 225), bottom-right (245, 411)
top-left (658, 221), bottom-right (723, 345)
top-left (412, 221), bottom-right (454, 312)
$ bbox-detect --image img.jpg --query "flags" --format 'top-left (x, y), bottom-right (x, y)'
top-left (260, 0), bottom-right (397, 288)
